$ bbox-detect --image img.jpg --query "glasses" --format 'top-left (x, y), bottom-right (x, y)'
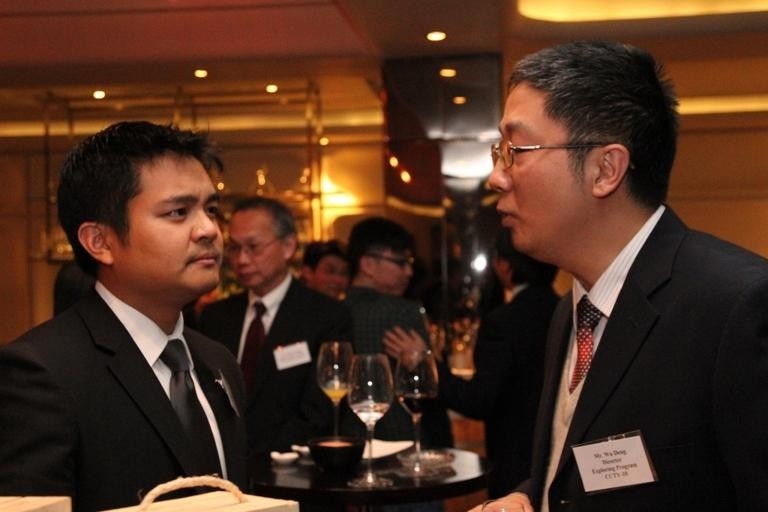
top-left (362, 249), bottom-right (416, 269)
top-left (489, 138), bottom-right (640, 174)
top-left (225, 236), bottom-right (282, 258)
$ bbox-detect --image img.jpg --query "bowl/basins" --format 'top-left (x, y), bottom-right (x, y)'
top-left (311, 439), bottom-right (365, 473)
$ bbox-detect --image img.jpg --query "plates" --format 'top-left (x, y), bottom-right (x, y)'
top-left (270, 451), bottom-right (300, 466)
top-left (290, 437), bottom-right (416, 465)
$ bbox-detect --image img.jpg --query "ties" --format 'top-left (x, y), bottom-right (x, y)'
top-left (570, 294), bottom-right (603, 393)
top-left (240, 300), bottom-right (267, 370)
top-left (159, 339), bottom-right (222, 479)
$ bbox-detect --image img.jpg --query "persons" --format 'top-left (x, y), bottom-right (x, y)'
top-left (380, 230), bottom-right (563, 497)
top-left (0, 119), bottom-right (249, 512)
top-left (52, 257), bottom-right (198, 329)
top-left (199, 194), bottom-right (355, 485)
top-left (464, 38), bottom-right (768, 512)
top-left (299, 238), bottom-right (352, 299)
top-left (342, 214), bottom-right (456, 450)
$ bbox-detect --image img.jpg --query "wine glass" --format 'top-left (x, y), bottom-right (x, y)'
top-left (346, 352), bottom-right (395, 489)
top-left (395, 348), bottom-right (441, 479)
top-left (315, 340), bottom-right (354, 436)
